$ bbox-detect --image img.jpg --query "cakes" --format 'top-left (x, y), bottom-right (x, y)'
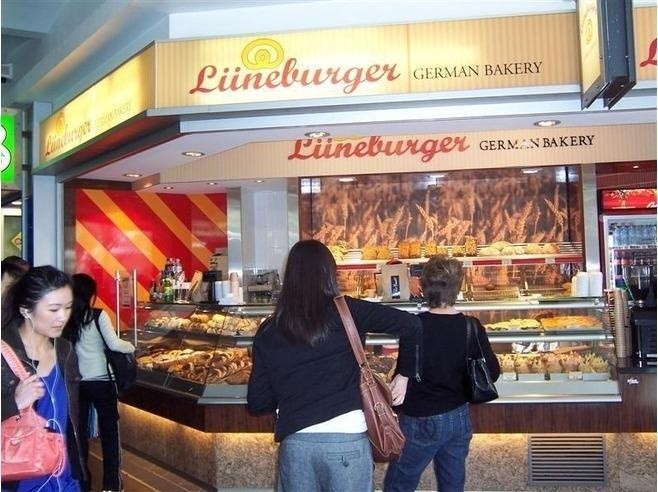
top-left (488, 315), bottom-right (607, 374)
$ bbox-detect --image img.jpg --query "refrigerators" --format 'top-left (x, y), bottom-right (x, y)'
top-left (596, 187), bottom-right (657, 301)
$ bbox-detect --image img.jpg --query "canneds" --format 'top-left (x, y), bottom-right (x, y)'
top-left (162, 258), bottom-right (182, 303)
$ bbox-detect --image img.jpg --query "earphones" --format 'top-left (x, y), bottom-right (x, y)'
top-left (24, 309), bottom-right (28, 314)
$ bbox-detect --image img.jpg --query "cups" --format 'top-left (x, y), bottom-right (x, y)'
top-left (214, 271), bottom-right (244, 304)
top-left (603, 288), bottom-right (632, 359)
top-left (571, 272), bottom-right (603, 297)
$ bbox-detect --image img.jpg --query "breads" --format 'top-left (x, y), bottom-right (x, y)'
top-left (361, 236), bottom-right (561, 260)
top-left (137, 313), bottom-right (259, 385)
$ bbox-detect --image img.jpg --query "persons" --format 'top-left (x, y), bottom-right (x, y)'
top-left (1, 265), bottom-right (93, 491)
top-left (245, 238), bottom-right (427, 491)
top-left (383, 252), bottom-right (500, 492)
top-left (2, 254), bottom-right (29, 304)
top-left (66, 273), bottom-right (139, 492)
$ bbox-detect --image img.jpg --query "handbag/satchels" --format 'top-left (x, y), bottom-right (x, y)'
top-left (105, 346), bottom-right (140, 398)
top-left (354, 363), bottom-right (407, 466)
top-left (462, 356), bottom-right (499, 408)
top-left (0, 408), bottom-right (69, 484)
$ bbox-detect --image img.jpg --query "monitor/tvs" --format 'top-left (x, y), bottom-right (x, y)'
top-left (576, 0), bottom-right (637, 111)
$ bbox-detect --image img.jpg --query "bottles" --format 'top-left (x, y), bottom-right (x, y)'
top-left (148, 257), bottom-right (187, 303)
top-left (379, 246), bottom-right (455, 302)
top-left (611, 222), bottom-right (657, 282)
top-left (249, 269), bottom-right (281, 304)
